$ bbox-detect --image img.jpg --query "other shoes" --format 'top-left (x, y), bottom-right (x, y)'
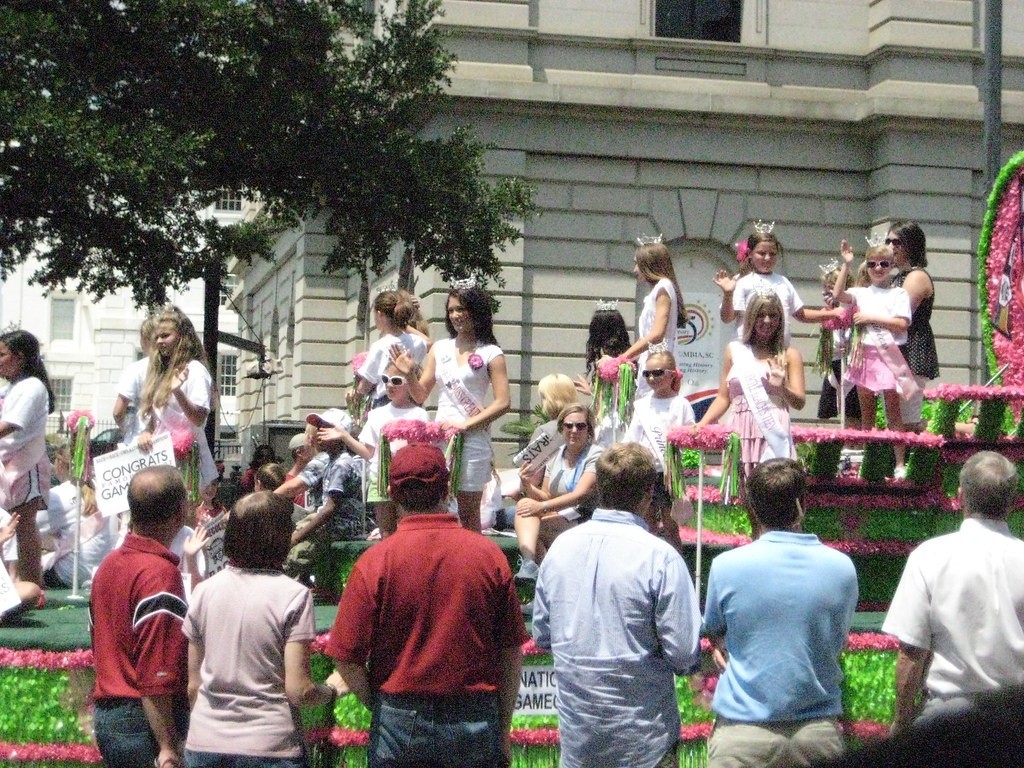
top-left (521, 634), bottom-right (544, 656)
top-left (519, 600), bottom-right (535, 615)
top-left (893, 467), bottom-right (908, 480)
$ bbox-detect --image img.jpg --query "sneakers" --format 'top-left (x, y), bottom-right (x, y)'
top-left (513, 554), bottom-right (538, 587)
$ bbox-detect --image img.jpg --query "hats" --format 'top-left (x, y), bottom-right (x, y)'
top-left (307, 408), bottom-right (352, 435)
top-left (390, 443), bottom-right (446, 492)
top-left (288, 433), bottom-right (307, 450)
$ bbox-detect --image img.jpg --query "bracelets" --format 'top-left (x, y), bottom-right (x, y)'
top-left (324, 683), bottom-right (337, 706)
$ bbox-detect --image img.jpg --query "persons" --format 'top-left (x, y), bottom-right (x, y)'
top-left (88, 465), bottom-right (190, 768)
top-left (702, 457), bottom-right (859, 768)
top-left (531, 442), bottom-right (702, 768)
top-left (819, 220), bottom-right (939, 479)
top-left (690, 291), bottom-right (806, 506)
top-left (388, 275), bottom-right (511, 534)
top-left (112, 305), bottom-right (226, 592)
top-left (239, 408), bottom-right (362, 609)
top-left (325, 442), bottom-right (532, 768)
top-left (315, 361), bottom-right (429, 541)
top-left (182, 490), bottom-right (349, 768)
top-left (882, 451), bottom-right (1024, 737)
top-left (504, 374), bottom-right (603, 590)
top-left (0, 330), bottom-right (55, 609)
top-left (713, 231), bottom-right (843, 348)
top-left (573, 298), bottom-right (632, 425)
top-left (620, 350), bottom-right (695, 473)
top-left (357, 288), bottom-right (432, 412)
top-left (36, 447), bottom-right (118, 591)
top-left (613, 232), bottom-right (690, 400)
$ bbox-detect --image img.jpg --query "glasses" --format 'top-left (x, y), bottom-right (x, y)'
top-left (885, 238), bottom-right (902, 245)
top-left (381, 374), bottom-right (407, 386)
top-left (642, 368), bottom-right (673, 378)
top-left (865, 260), bottom-right (892, 269)
top-left (563, 422), bottom-right (588, 431)
